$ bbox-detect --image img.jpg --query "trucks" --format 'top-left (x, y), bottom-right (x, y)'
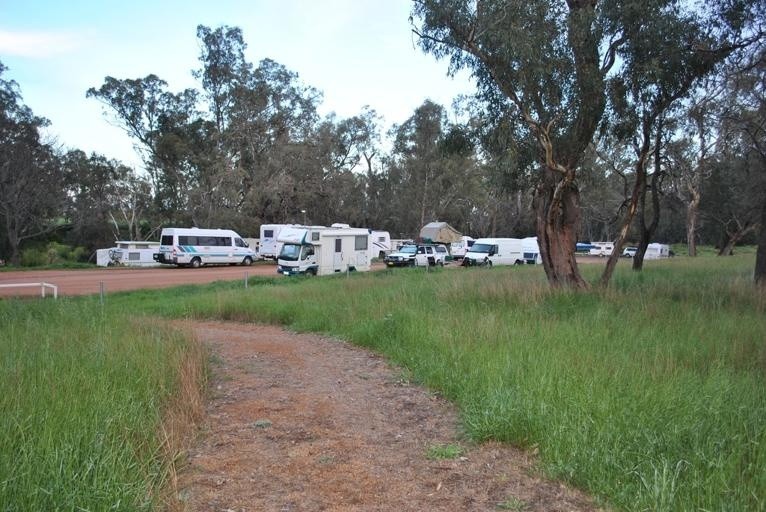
top-left (588, 241), bottom-right (616, 258)
top-left (645, 243), bottom-right (671, 260)
top-left (152, 223), bottom-right (412, 277)
top-left (445, 232), bottom-right (543, 266)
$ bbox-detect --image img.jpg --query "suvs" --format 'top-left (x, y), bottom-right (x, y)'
top-left (384, 242), bottom-right (451, 271)
top-left (623, 247), bottom-right (639, 257)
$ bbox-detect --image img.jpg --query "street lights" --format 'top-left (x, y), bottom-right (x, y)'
top-left (301, 209), bottom-right (309, 226)
